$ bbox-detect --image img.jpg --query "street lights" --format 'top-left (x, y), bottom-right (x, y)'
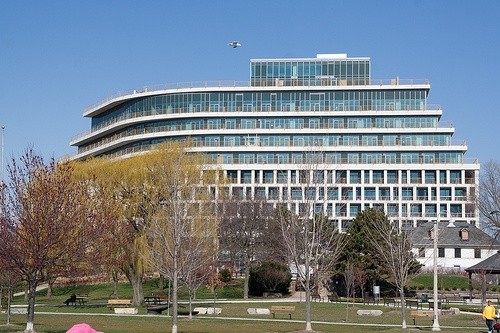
top-left (426, 223), bottom-right (442, 332)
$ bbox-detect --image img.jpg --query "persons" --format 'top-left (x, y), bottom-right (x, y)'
top-left (63, 294), bottom-right (76, 306)
top-left (482, 301), bottom-right (496, 333)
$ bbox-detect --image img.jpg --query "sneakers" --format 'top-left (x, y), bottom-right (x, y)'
top-left (488, 330), bottom-right (492, 333)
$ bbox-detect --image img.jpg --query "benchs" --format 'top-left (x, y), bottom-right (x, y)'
top-left (410, 310), bottom-right (439, 326)
top-left (66, 296), bottom-right (88, 306)
top-left (405, 299), bottom-right (423, 310)
top-left (428, 300), bottom-right (447, 310)
top-left (383, 298), bottom-right (399, 308)
top-left (310, 294), bottom-right (325, 302)
top-left (106, 299), bottom-right (132, 310)
top-left (327, 294), bottom-right (342, 303)
top-left (142, 295), bottom-right (162, 304)
top-left (271, 305), bottom-right (296, 318)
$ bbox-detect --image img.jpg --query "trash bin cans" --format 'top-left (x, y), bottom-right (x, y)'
top-left (422, 293), bottom-right (428, 303)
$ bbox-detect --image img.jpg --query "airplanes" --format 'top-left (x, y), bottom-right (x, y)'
top-left (227, 40), bottom-right (241, 49)
